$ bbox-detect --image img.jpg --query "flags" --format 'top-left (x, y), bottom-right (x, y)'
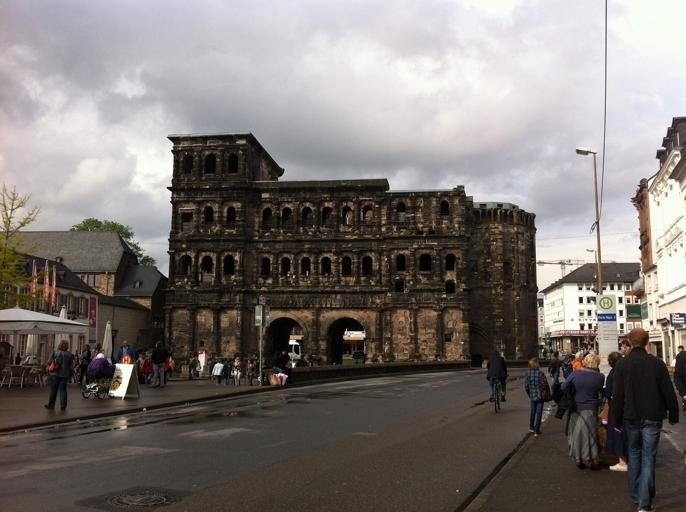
top-left (44, 260), bottom-right (49, 303)
top-left (31, 259), bottom-right (38, 300)
top-left (52, 266), bottom-right (57, 308)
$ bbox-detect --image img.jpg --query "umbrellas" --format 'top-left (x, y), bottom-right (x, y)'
top-left (53, 304), bottom-right (70, 350)
top-left (0, 306), bottom-right (88, 365)
top-left (25, 333), bottom-right (41, 364)
top-left (101, 319), bottom-right (114, 366)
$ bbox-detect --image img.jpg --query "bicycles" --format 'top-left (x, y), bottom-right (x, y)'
top-left (489, 376), bottom-right (504, 414)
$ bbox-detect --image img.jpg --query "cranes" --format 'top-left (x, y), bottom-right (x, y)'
top-left (536, 258), bottom-right (618, 277)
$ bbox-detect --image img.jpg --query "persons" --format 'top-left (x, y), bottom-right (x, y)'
top-left (42, 339), bottom-right (74, 411)
top-left (673, 345), bottom-right (686, 411)
top-left (612, 327), bottom-right (680, 512)
top-left (524, 338), bottom-right (632, 472)
top-left (486, 349), bottom-right (507, 402)
top-left (14, 352), bottom-right (21, 365)
top-left (73, 339), bottom-right (172, 389)
top-left (208, 348), bottom-right (291, 387)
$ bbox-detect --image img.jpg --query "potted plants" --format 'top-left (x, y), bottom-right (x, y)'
top-left (267, 366), bottom-right (287, 386)
top-left (311, 354), bottom-right (320, 367)
top-left (352, 350), bottom-right (368, 364)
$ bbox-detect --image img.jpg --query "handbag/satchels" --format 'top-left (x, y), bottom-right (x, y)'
top-left (49, 359), bottom-right (59, 371)
top-left (540, 375), bottom-right (552, 403)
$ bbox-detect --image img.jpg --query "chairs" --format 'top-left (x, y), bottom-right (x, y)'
top-left (0, 363), bottom-right (81, 390)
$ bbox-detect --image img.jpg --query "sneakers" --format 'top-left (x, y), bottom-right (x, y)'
top-left (500, 396), bottom-right (506, 402)
top-left (489, 397), bottom-right (494, 402)
top-left (575, 458), bottom-right (585, 467)
top-left (591, 461), bottom-right (600, 470)
top-left (529, 428), bottom-right (534, 432)
top-left (534, 433), bottom-right (542, 437)
top-left (44, 405), bottom-right (55, 409)
top-left (61, 407), bottom-right (66, 411)
top-left (632, 498), bottom-right (656, 512)
top-left (609, 463), bottom-right (627, 471)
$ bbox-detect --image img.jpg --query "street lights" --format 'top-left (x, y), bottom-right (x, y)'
top-left (573, 144), bottom-right (604, 296)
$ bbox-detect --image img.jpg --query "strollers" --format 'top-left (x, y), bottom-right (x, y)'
top-left (79, 353), bottom-right (114, 400)
